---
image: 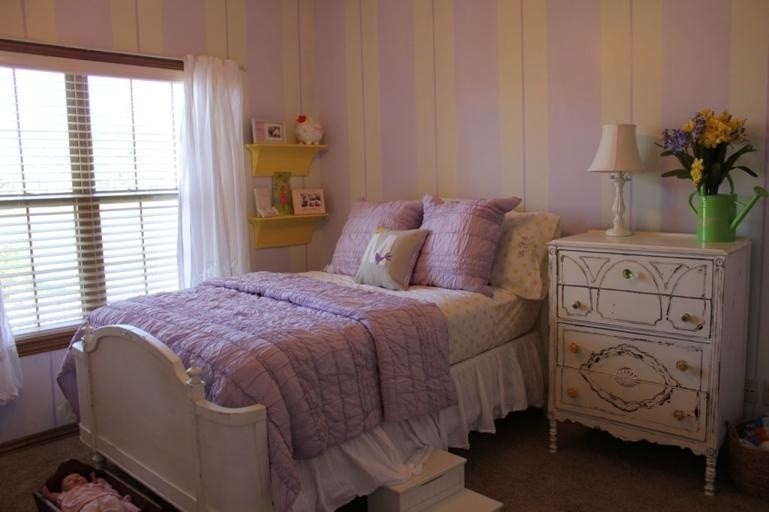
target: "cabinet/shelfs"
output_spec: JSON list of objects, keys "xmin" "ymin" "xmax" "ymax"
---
[{"xmin": 243, "ymin": 143, "xmax": 329, "ymax": 250}]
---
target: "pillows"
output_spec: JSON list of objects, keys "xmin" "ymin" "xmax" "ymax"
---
[{"xmin": 327, "ymin": 193, "xmax": 561, "ymax": 301}]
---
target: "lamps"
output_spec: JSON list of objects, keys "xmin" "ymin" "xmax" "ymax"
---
[{"xmin": 587, "ymin": 123, "xmax": 645, "ymax": 237}]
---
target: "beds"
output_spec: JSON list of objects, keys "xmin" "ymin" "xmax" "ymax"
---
[
  {"xmin": 72, "ymin": 270, "xmax": 549, "ymax": 512},
  {"xmin": 33, "ymin": 457, "xmax": 165, "ymax": 512}
]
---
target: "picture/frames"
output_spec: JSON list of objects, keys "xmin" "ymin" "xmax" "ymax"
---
[
  {"xmin": 291, "ymin": 189, "xmax": 326, "ymax": 215},
  {"xmin": 251, "ymin": 117, "xmax": 287, "ymax": 144}
]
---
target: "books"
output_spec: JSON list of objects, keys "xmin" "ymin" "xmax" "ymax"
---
[{"xmin": 253, "ymin": 187, "xmax": 272, "ymax": 219}]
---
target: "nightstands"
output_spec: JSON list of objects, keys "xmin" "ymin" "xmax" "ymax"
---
[{"xmin": 546, "ymin": 230, "xmax": 753, "ymax": 497}]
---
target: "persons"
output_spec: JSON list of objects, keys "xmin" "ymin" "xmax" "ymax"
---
[
  {"xmin": 39, "ymin": 470, "xmax": 142, "ymax": 512},
  {"xmin": 301, "ymin": 194, "xmax": 320, "ymax": 207},
  {"xmin": 272, "ymin": 126, "xmax": 280, "ymax": 137}
]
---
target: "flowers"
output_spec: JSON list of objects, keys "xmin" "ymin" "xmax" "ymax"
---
[{"xmin": 654, "ymin": 108, "xmax": 758, "ymax": 194}]
